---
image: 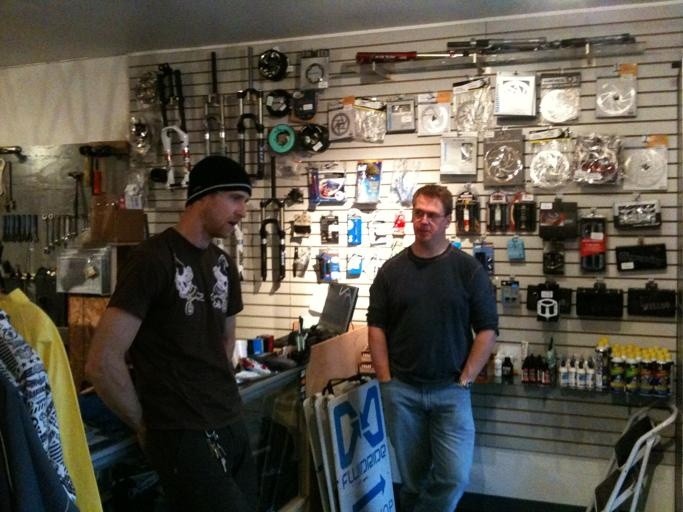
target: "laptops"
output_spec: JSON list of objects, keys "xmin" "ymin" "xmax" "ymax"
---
[{"xmin": 275, "ymin": 282, "xmax": 359, "ymax": 347}]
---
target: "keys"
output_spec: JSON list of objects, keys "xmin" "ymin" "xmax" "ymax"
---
[{"xmin": 205, "ymin": 428, "xmax": 228, "ymax": 473}]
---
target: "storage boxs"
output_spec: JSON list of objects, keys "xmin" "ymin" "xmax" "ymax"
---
[{"xmin": 89, "ymin": 208, "xmax": 143, "ymax": 242}]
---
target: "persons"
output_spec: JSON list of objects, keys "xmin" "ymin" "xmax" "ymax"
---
[
  {"xmin": 84, "ymin": 156, "xmax": 259, "ymax": 512},
  {"xmin": 365, "ymin": 185, "xmax": 499, "ymax": 512}
]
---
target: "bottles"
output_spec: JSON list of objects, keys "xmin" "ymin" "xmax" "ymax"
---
[
  {"xmin": 493, "ymin": 355, "xmax": 514, "ymax": 378},
  {"xmin": 519, "ymin": 347, "xmax": 594, "ymax": 392},
  {"xmin": 593, "ymin": 336, "xmax": 676, "ymax": 397},
  {"xmin": 295, "ymin": 333, "xmax": 304, "ymax": 351}
]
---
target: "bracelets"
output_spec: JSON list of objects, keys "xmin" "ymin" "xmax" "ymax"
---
[{"xmin": 461, "ymin": 380, "xmax": 473, "ymax": 390}]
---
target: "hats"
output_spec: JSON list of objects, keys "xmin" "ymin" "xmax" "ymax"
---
[{"xmin": 185, "ymin": 155, "xmax": 251, "ymax": 209}]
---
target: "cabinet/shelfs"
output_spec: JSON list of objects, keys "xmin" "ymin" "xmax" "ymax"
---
[{"xmin": 78, "ymin": 363, "xmax": 312, "ymax": 512}]
